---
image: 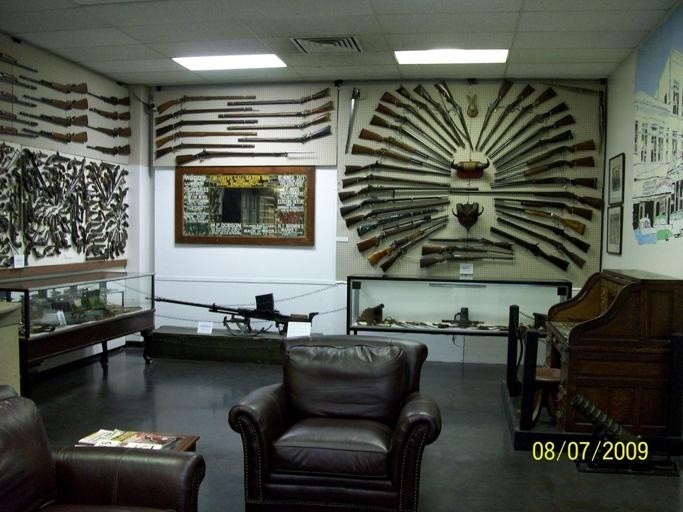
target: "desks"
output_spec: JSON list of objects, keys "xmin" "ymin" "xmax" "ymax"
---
[
  {"xmin": 152, "ymin": 429, "xmax": 200, "ymax": 456},
  {"xmin": 546, "ymin": 268, "xmax": 683, "ymax": 432}
]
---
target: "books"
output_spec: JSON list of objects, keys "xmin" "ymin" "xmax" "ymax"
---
[{"xmin": 75, "ymin": 429, "xmax": 185, "ymax": 450}]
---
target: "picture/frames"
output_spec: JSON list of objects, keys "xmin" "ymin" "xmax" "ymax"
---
[
  {"xmin": 171, "ymin": 166, "xmax": 317, "ymax": 251},
  {"xmin": 606, "ymin": 153, "xmax": 625, "ymax": 255}
]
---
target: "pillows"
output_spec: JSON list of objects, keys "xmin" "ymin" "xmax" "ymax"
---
[{"xmin": 273, "ymin": 416, "xmax": 396, "ymax": 474}]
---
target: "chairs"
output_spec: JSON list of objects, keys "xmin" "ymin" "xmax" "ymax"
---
[
  {"xmin": 227, "ymin": 332, "xmax": 444, "ymax": 512},
  {"xmin": 0, "ymin": 385, "xmax": 205, "ymax": 511}
]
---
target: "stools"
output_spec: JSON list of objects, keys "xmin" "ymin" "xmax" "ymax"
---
[{"xmin": 533, "ymin": 367, "xmax": 562, "ymax": 425}]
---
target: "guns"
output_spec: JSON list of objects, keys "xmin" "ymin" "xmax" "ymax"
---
[
  {"xmin": 145, "ymin": 292, "xmax": 319, "ymax": 333},
  {"xmin": 86, "ymin": 89, "xmax": 131, "ymax": 139},
  {"xmin": 155, "ymin": 85, "xmax": 334, "ymax": 165},
  {"xmin": 19, "ymin": 74, "xmax": 88, "ymax": 145},
  {"xmin": 0, "ymin": 51, "xmax": 36, "ymax": 139},
  {"xmin": 0, "ymin": 140, "xmax": 130, "ymax": 269}
]
